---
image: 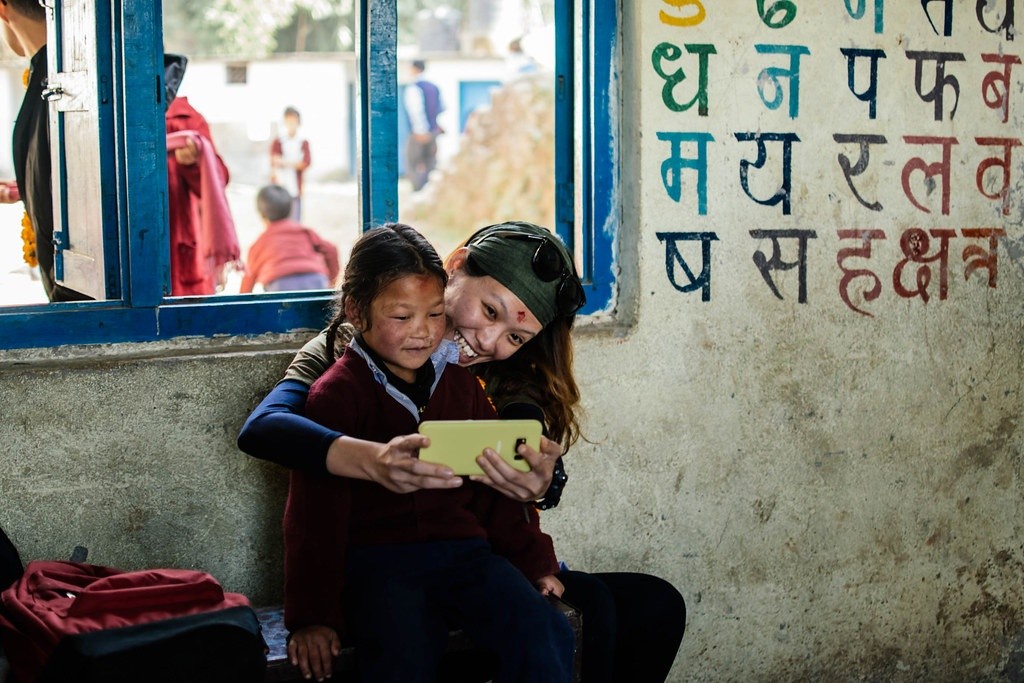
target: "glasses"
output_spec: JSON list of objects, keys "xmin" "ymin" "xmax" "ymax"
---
[{"xmin": 471, "ymin": 225, "xmax": 586, "ymax": 323}]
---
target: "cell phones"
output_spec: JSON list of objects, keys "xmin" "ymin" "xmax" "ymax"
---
[{"xmin": 417, "ymin": 419, "xmax": 542, "ymax": 476}]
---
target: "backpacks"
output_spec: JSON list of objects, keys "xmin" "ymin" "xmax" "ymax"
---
[{"xmin": 0, "ymin": 532, "xmax": 270, "ymax": 681}]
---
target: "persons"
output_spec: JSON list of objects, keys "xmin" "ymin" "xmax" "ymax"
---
[
  {"xmin": 0, "ymin": 1, "xmax": 90, "ymax": 307},
  {"xmin": 164, "ymin": 95, "xmax": 239, "ymax": 298},
  {"xmin": 403, "ymin": 59, "xmax": 445, "ymax": 192},
  {"xmin": 236, "ymin": 221, "xmax": 687, "ymax": 683},
  {"xmin": 285, "ymin": 223, "xmax": 578, "ymax": 683},
  {"xmin": 270, "ymin": 106, "xmax": 311, "ymax": 223},
  {"xmin": 238, "ymin": 184, "xmax": 339, "ymax": 296}
]
